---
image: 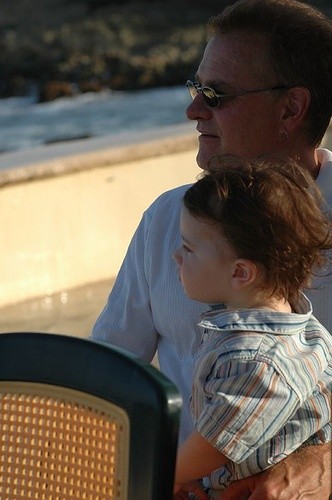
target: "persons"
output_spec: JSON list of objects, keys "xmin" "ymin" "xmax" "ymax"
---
[
  {"xmin": 87, "ymin": 1, "xmax": 332, "ymax": 500},
  {"xmin": 174, "ymin": 153, "xmax": 332, "ymax": 500}
]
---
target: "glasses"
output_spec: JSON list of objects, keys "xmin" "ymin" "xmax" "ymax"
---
[{"xmin": 184, "ymin": 80, "xmax": 320, "ymax": 110}]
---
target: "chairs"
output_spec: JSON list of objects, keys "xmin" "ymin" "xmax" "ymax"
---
[{"xmin": 0, "ymin": 332, "xmax": 182, "ymax": 500}]
